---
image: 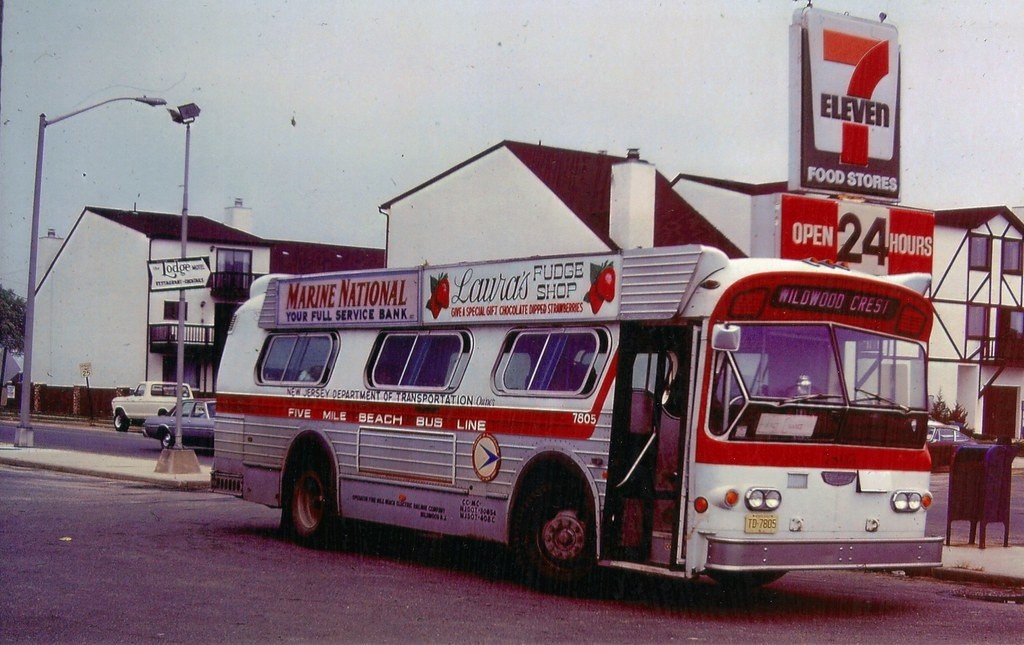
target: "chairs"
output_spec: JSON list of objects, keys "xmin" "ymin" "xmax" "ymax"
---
[{"xmin": 630, "ymin": 388, "xmax": 653, "ymax": 433}]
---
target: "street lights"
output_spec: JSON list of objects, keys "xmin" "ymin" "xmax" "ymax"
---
[
  {"xmin": 13, "ymin": 95, "xmax": 169, "ymax": 447},
  {"xmin": 165, "ymin": 101, "xmax": 205, "ymax": 448}
]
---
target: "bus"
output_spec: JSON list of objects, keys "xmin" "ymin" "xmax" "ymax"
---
[{"xmin": 207, "ymin": 246, "xmax": 944, "ymax": 593}]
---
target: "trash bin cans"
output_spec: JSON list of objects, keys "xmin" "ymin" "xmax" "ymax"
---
[
  {"xmin": 947, "ymin": 443, "xmax": 1020, "ymax": 548},
  {"xmin": 6, "ymin": 381, "xmax": 22, "ymax": 413}
]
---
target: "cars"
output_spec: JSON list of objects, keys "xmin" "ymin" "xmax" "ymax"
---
[
  {"xmin": 144, "ymin": 397, "xmax": 214, "ymax": 449},
  {"xmin": 927, "ymin": 423, "xmax": 980, "ymax": 471},
  {"xmin": 109, "ymin": 382, "xmax": 195, "ymax": 433}
]
